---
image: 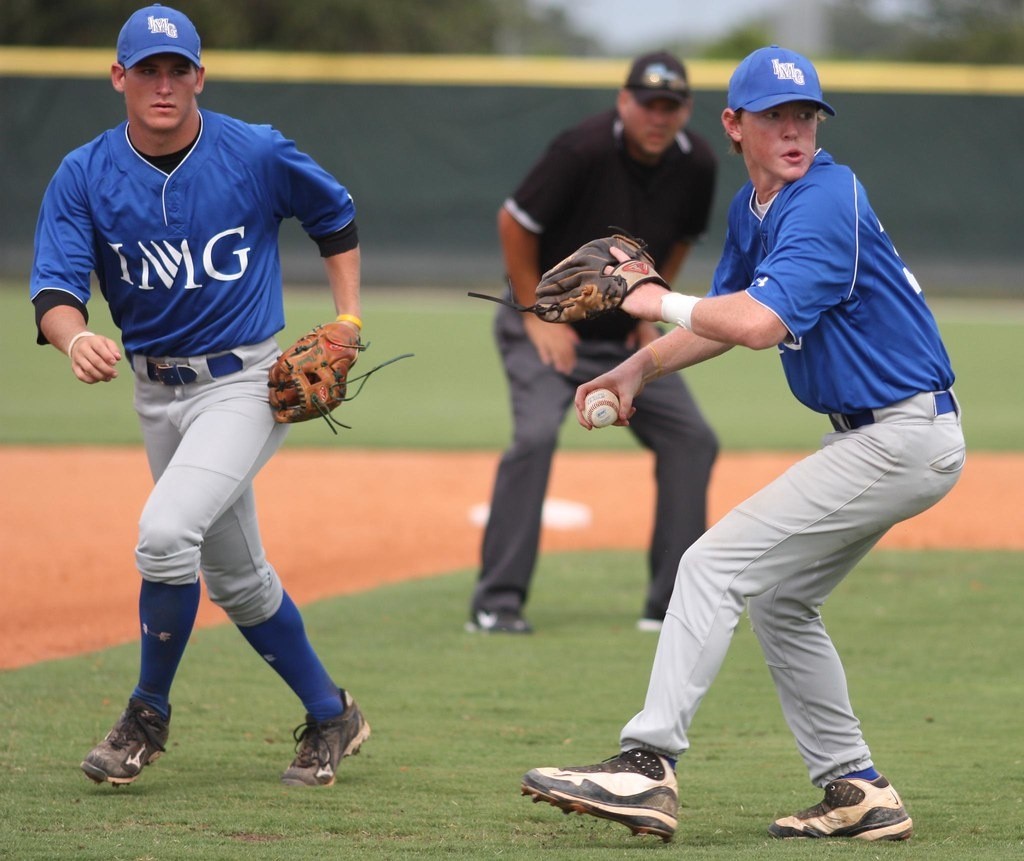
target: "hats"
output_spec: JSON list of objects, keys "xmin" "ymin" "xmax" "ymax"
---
[
  {"xmin": 117, "ymin": 3, "xmax": 201, "ymax": 70},
  {"xmin": 728, "ymin": 44, "xmax": 836, "ymax": 117},
  {"xmin": 624, "ymin": 51, "xmax": 690, "ymax": 109}
]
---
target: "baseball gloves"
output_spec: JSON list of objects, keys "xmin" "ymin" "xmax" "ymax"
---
[
  {"xmin": 266, "ymin": 323, "xmax": 360, "ymax": 424},
  {"xmin": 536, "ymin": 233, "xmax": 672, "ymax": 322}
]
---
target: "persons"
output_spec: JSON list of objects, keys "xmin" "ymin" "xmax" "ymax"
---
[
  {"xmin": 466, "ymin": 50, "xmax": 720, "ymax": 637},
  {"xmin": 29, "ymin": 2, "xmax": 371, "ymax": 786},
  {"xmin": 522, "ymin": 45, "xmax": 964, "ymax": 839}
]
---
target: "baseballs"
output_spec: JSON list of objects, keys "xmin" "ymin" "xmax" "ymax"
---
[{"xmin": 581, "ymin": 388, "xmax": 621, "ymax": 430}]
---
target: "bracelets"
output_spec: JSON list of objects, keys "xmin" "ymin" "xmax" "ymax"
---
[
  {"xmin": 67, "ymin": 331, "xmax": 96, "ymax": 360},
  {"xmin": 646, "ymin": 344, "xmax": 661, "ymax": 380},
  {"xmin": 335, "ymin": 314, "xmax": 362, "ymax": 331}
]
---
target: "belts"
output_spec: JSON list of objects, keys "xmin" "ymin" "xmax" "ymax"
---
[
  {"xmin": 131, "ymin": 352, "xmax": 242, "ymax": 386},
  {"xmin": 828, "ymin": 390, "xmax": 955, "ymax": 431}
]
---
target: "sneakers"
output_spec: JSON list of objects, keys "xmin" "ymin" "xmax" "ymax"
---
[
  {"xmin": 281, "ymin": 687, "xmax": 371, "ymax": 789},
  {"xmin": 520, "ymin": 749, "xmax": 679, "ymax": 843},
  {"xmin": 767, "ymin": 770, "xmax": 913, "ymax": 842},
  {"xmin": 80, "ymin": 696, "xmax": 172, "ymax": 788},
  {"xmin": 464, "ymin": 607, "xmax": 532, "ymax": 636},
  {"xmin": 638, "ymin": 605, "xmax": 664, "ymax": 632}
]
